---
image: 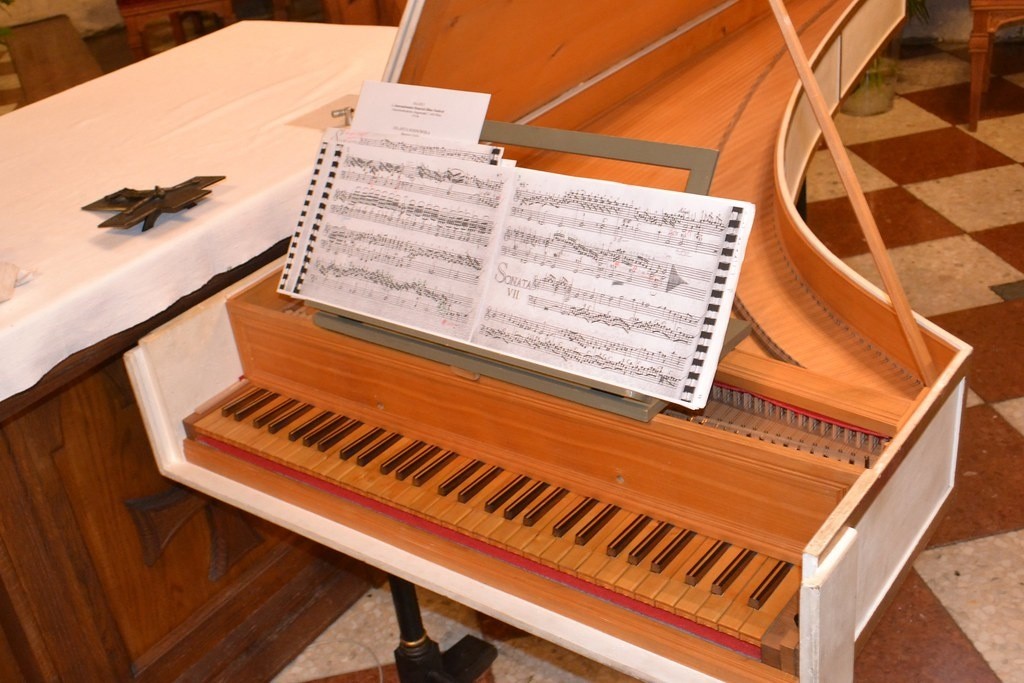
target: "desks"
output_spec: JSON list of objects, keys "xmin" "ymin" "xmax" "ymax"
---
[{"xmin": 0, "ymin": 16, "xmax": 403, "ymax": 682}]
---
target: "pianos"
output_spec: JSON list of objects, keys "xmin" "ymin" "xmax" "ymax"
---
[{"xmin": 122, "ymin": 0, "xmax": 973, "ymax": 683}]
